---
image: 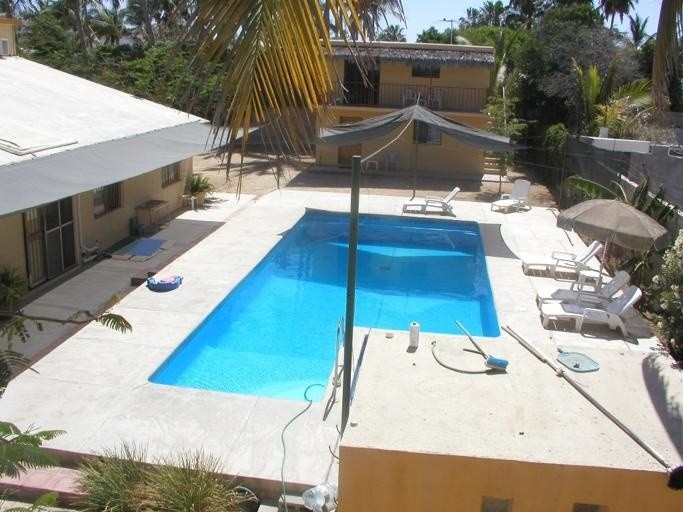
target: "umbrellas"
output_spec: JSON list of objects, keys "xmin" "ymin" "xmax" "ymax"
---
[{"xmin": 557, "ymin": 198, "xmax": 669, "ymax": 287}]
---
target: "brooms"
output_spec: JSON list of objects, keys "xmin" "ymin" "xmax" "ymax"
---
[{"xmin": 454, "ymin": 320, "xmax": 509, "ymax": 370}]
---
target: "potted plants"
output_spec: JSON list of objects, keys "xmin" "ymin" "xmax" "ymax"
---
[{"xmin": 189, "ymin": 175, "xmax": 212, "ymax": 207}]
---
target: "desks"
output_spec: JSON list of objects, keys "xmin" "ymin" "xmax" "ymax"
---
[
  {"xmin": 135, "ymin": 200, "xmax": 172, "ymax": 235},
  {"xmin": 577, "ymin": 270, "xmax": 603, "ymax": 292}
]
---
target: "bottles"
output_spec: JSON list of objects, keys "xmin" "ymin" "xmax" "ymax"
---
[{"xmin": 409, "ymin": 323, "xmax": 419, "ymax": 348}]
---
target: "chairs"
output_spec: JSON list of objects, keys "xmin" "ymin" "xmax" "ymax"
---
[
  {"xmin": 521, "ymin": 240, "xmax": 604, "ymax": 282},
  {"xmin": 491, "ymin": 179, "xmax": 532, "ymax": 213},
  {"xmin": 365, "ymin": 159, "xmax": 380, "ymax": 170},
  {"xmin": 539, "ymin": 285, "xmax": 643, "ymax": 336},
  {"xmin": 410, "ymin": 229, "xmax": 457, "ymax": 251},
  {"xmin": 536, "ymin": 270, "xmax": 631, "ymax": 310},
  {"xmin": 403, "ymin": 186, "xmax": 460, "ymax": 217},
  {"xmin": 386, "ymin": 150, "xmax": 401, "ymax": 172}
]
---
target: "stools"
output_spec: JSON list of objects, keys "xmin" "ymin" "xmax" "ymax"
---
[{"xmin": 178, "ymin": 194, "xmax": 199, "ymax": 210}]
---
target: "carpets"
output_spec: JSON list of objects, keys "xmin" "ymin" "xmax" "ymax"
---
[{"xmin": 104, "ymin": 232, "xmax": 167, "ymax": 257}]
